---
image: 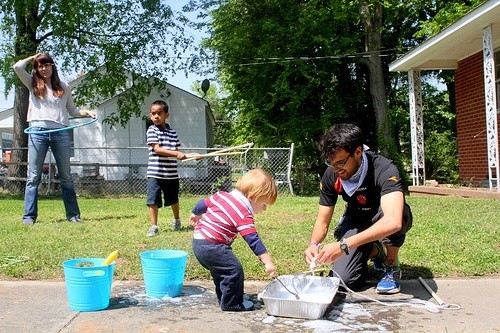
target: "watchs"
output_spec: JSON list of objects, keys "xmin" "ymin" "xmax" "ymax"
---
[{"xmin": 340, "ymin": 239, "xmax": 349, "ymax": 255}]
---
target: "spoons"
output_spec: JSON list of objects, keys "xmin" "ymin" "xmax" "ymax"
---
[{"xmin": 276, "ymin": 276, "xmax": 299, "ymax": 300}]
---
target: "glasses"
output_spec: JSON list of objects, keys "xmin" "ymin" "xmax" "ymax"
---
[
  {"xmin": 324, "ymin": 150, "xmax": 351, "ymax": 169},
  {"xmin": 36, "ymin": 63, "xmax": 55, "ymax": 68}
]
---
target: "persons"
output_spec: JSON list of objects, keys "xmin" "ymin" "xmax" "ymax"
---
[
  {"xmin": 189, "ymin": 168, "xmax": 278, "ymax": 311},
  {"xmin": 146, "ymin": 100, "xmax": 202, "ymax": 236},
  {"xmin": 304, "ymin": 123, "xmax": 413, "ymax": 294},
  {"xmin": 14, "ymin": 53, "xmax": 98, "ymax": 224}
]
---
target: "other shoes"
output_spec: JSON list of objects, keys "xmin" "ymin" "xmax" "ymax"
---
[
  {"xmin": 22, "ymin": 219, "xmax": 36, "ymax": 226},
  {"xmin": 370, "ymin": 241, "xmax": 386, "ymax": 277},
  {"xmin": 220, "ymin": 300, "xmax": 254, "ymax": 311},
  {"xmin": 70, "ymin": 215, "xmax": 82, "ymax": 223},
  {"xmin": 216, "ymin": 295, "xmax": 250, "ymax": 302},
  {"xmin": 376, "ymin": 263, "xmax": 403, "ymax": 293},
  {"xmin": 146, "ymin": 226, "xmax": 159, "ymax": 237},
  {"xmin": 173, "ymin": 222, "xmax": 181, "ymax": 230}
]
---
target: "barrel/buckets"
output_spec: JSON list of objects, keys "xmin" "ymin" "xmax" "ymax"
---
[
  {"xmin": 140, "ymin": 249, "xmax": 188, "ymax": 299},
  {"xmin": 62, "ymin": 257, "xmax": 115, "ymax": 312}
]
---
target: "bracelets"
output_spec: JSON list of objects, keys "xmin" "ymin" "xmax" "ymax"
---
[{"xmin": 310, "ymin": 243, "xmax": 321, "ymax": 248}]
